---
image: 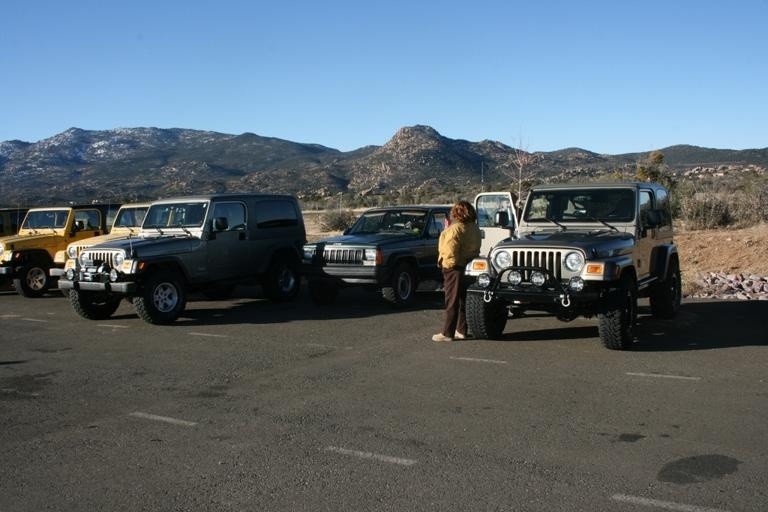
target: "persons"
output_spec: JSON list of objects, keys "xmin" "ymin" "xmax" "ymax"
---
[{"xmin": 432, "ymin": 200, "xmax": 482, "ymax": 343}]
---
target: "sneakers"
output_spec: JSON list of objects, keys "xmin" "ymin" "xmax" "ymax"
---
[
  {"xmin": 454, "ymin": 330, "xmax": 466, "ymax": 339},
  {"xmin": 432, "ymin": 333, "xmax": 453, "ymax": 342}
]
